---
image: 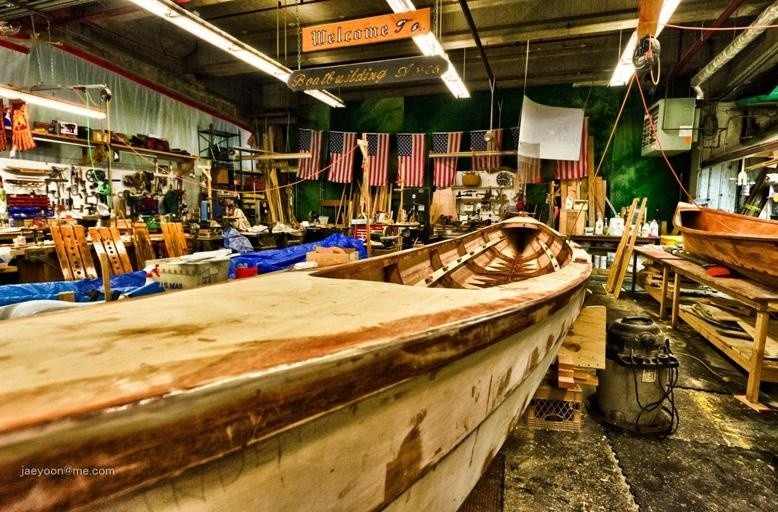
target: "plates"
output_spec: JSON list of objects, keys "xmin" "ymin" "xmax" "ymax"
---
[{"xmin": 497, "ymin": 173, "xmax": 510, "ymax": 185}]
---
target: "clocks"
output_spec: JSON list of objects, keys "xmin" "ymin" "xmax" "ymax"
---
[{"xmin": 496, "ymin": 172, "xmax": 511, "ymax": 186}]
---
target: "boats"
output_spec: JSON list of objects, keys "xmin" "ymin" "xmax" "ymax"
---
[
  {"xmin": 0, "ymin": 215, "xmax": 593, "ymax": 511},
  {"xmin": 672, "ymin": 202, "xmax": 778, "ymax": 289}
]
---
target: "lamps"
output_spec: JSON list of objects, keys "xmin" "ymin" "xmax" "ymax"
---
[
  {"xmin": 385, "ymin": 0, "xmax": 473, "ymax": 100},
  {"xmin": 128, "ymin": 0, "xmax": 347, "ymax": 109},
  {"xmin": 0, "ymin": 82, "xmax": 107, "ymax": 119},
  {"xmin": 608, "ymin": 0, "xmax": 682, "ymax": 88}
]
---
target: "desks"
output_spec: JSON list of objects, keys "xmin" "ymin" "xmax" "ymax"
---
[
  {"xmin": 662, "ymin": 247, "xmax": 778, "ymax": 403},
  {"xmin": 627, "ymin": 245, "xmax": 688, "ymax": 319},
  {"xmin": 571, "ymin": 235, "xmax": 660, "ymax": 292},
  {"xmin": 0, "ymin": 228, "xmax": 350, "ymax": 286}
]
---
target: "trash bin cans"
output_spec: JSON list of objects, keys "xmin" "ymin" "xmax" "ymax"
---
[{"xmin": 305, "ymin": 228, "xmax": 322, "ymax": 243}]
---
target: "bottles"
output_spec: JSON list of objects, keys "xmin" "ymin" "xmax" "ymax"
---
[
  {"xmin": 595, "ymin": 212, "xmax": 609, "ymax": 236},
  {"xmin": 642, "ymin": 218, "xmax": 658, "ymax": 237},
  {"xmin": 462, "ymin": 173, "xmax": 480, "ymax": 187}
]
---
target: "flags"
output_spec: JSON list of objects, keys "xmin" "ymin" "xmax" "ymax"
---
[
  {"xmin": 294, "ymin": 127, "xmax": 323, "ymax": 181},
  {"xmin": 396, "ymin": 132, "xmax": 426, "ymax": 186},
  {"xmin": 471, "ymin": 129, "xmax": 503, "ymax": 172},
  {"xmin": 555, "ymin": 117, "xmax": 590, "ymax": 182},
  {"xmin": 431, "ymin": 129, "xmax": 465, "ymax": 187},
  {"xmin": 509, "ymin": 126, "xmax": 543, "ymax": 185},
  {"xmin": 360, "ymin": 132, "xmax": 390, "ymax": 187},
  {"xmin": 326, "ymin": 130, "xmax": 356, "ymax": 184}
]
